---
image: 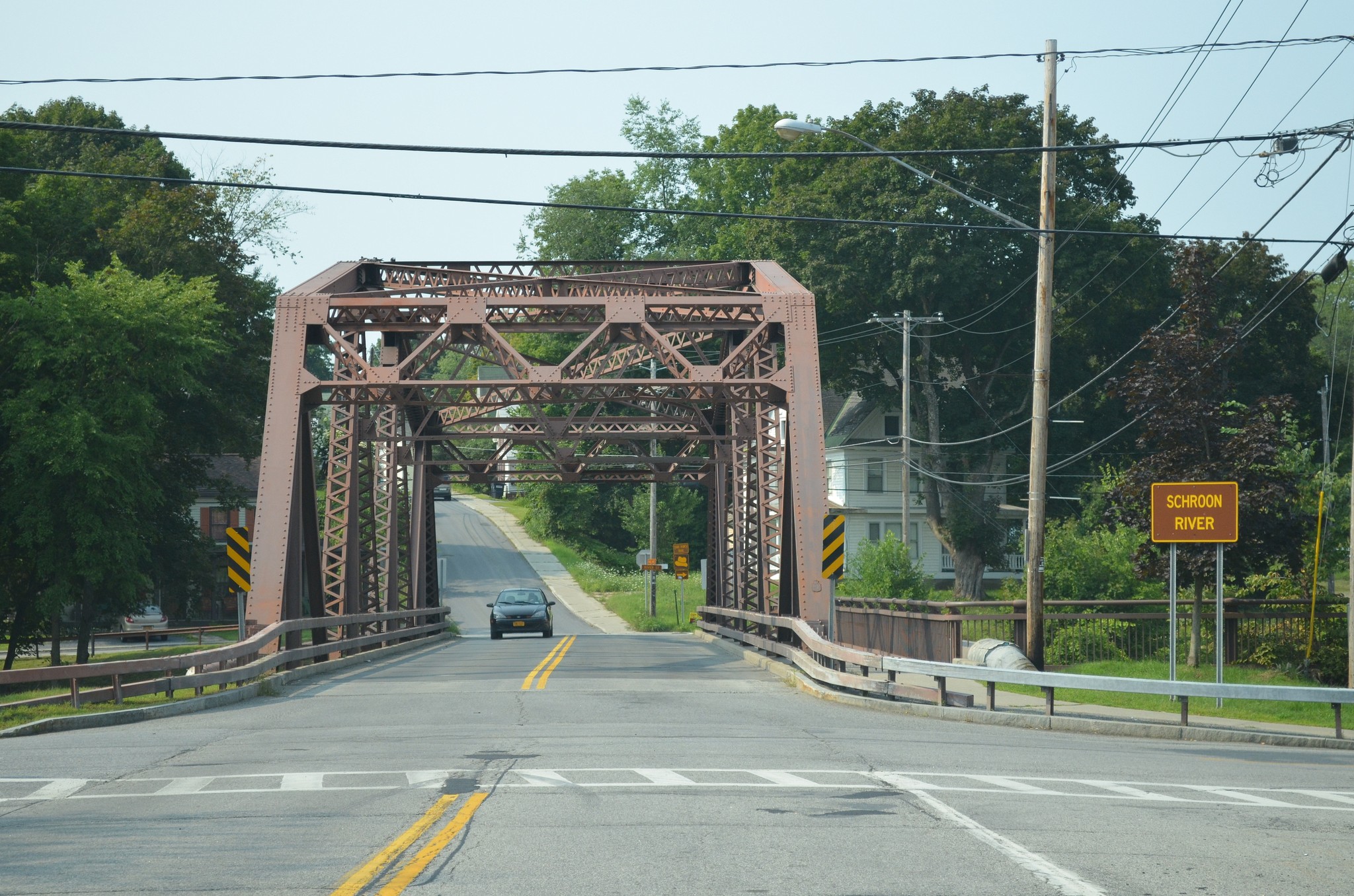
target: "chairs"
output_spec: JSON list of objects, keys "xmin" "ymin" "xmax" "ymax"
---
[
  {"xmin": 528, "ymin": 595, "xmax": 538, "ymax": 601},
  {"xmin": 506, "ymin": 596, "xmax": 515, "ymax": 603}
]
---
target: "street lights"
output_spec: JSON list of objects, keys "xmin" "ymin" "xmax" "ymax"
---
[{"xmin": 774, "ymin": 117, "xmax": 1058, "ymax": 670}]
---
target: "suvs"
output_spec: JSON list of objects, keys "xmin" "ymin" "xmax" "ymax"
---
[{"xmin": 434, "ymin": 475, "xmax": 452, "ymax": 501}]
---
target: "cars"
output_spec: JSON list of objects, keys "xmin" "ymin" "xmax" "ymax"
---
[
  {"xmin": 120, "ymin": 604, "xmax": 168, "ymax": 642},
  {"xmin": 486, "ymin": 587, "xmax": 555, "ymax": 639}
]
